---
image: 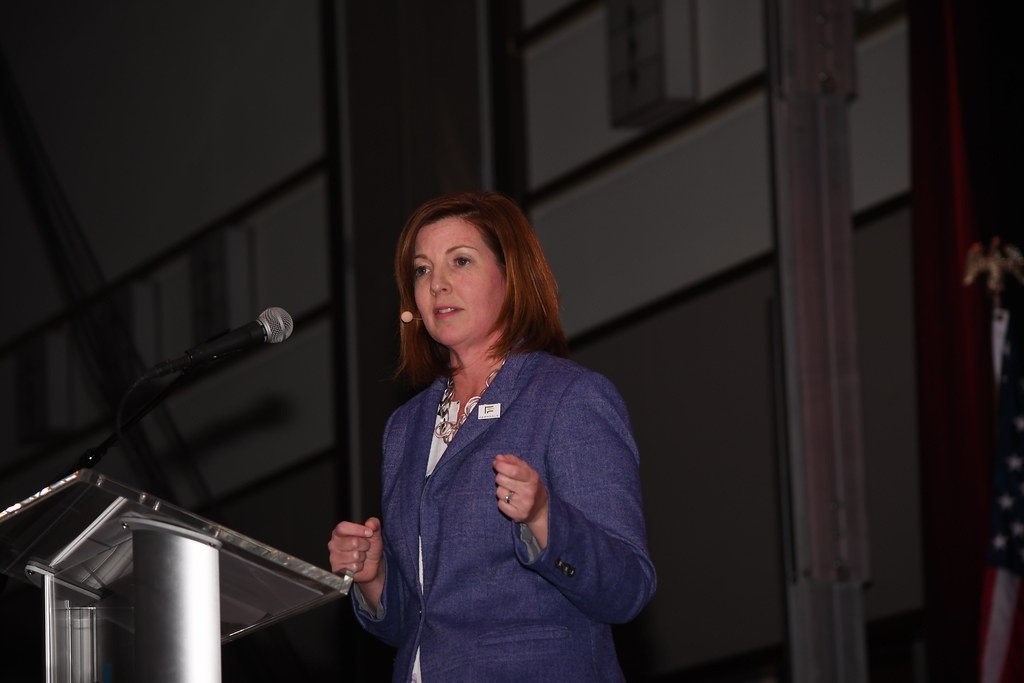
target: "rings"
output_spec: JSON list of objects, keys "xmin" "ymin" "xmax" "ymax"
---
[{"xmin": 504, "ymin": 491, "xmax": 516, "ymax": 504}]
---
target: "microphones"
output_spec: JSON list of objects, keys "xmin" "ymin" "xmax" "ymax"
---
[
  {"xmin": 401, "ymin": 311, "xmax": 423, "ymax": 322},
  {"xmin": 143, "ymin": 306, "xmax": 293, "ymax": 380}
]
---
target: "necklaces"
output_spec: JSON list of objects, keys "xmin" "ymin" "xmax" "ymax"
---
[{"xmin": 432, "ymin": 359, "xmax": 509, "ymax": 445}]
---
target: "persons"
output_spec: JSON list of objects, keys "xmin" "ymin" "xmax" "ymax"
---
[{"xmin": 328, "ymin": 190, "xmax": 662, "ymax": 683}]
